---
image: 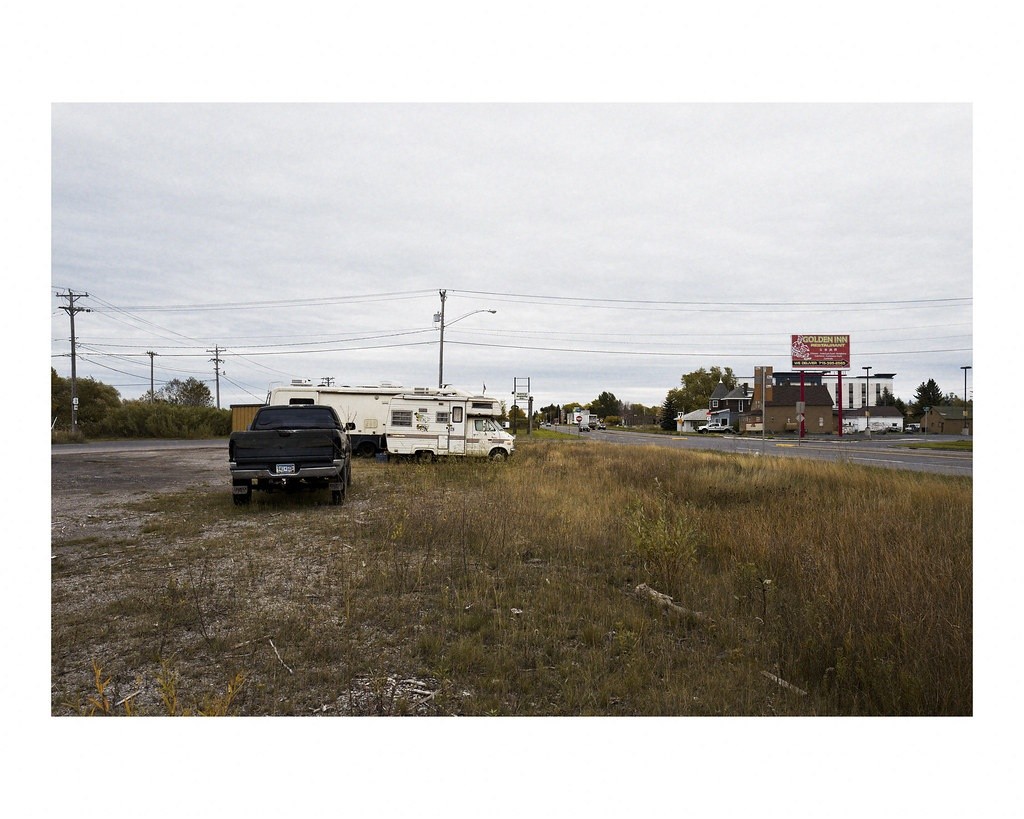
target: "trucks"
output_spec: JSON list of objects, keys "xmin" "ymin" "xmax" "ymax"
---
[
  {"xmin": 579, "ymin": 414, "xmax": 598, "ymax": 430},
  {"xmin": 268, "ymin": 385, "xmax": 474, "ymax": 459},
  {"xmin": 386, "ymin": 394, "xmax": 515, "ymax": 462}
]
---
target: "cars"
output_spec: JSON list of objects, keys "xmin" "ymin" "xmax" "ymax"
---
[
  {"xmin": 578, "ymin": 420, "xmax": 607, "ymax": 432},
  {"xmin": 905, "ymin": 423, "xmax": 920, "ymax": 431},
  {"xmin": 546, "ymin": 423, "xmax": 552, "ymax": 427}
]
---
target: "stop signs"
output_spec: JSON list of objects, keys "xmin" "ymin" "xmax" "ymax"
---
[{"xmin": 576, "ymin": 416, "xmax": 583, "ymax": 423}]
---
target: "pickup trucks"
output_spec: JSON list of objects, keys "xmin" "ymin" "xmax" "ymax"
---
[
  {"xmin": 229, "ymin": 405, "xmax": 356, "ymax": 506},
  {"xmin": 696, "ymin": 422, "xmax": 734, "ymax": 435}
]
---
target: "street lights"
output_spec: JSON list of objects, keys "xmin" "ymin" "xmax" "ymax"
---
[
  {"xmin": 438, "ymin": 310, "xmax": 498, "ymax": 388},
  {"xmin": 959, "ymin": 366, "xmax": 973, "ymax": 435},
  {"xmin": 862, "ymin": 366, "xmax": 873, "ymax": 440}
]
